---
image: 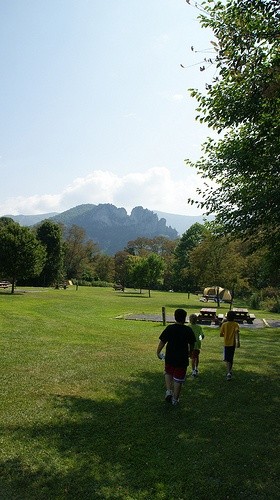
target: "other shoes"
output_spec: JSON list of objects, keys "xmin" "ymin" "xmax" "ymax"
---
[
  {"xmin": 165, "ymin": 390, "xmax": 179, "ymax": 406},
  {"xmin": 191, "ymin": 368, "xmax": 198, "ymax": 377},
  {"xmin": 226, "ymin": 371, "xmax": 232, "ymax": 381}
]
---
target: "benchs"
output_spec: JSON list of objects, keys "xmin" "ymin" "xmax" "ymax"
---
[{"xmin": 194, "ymin": 313, "xmax": 256, "ymax": 320}]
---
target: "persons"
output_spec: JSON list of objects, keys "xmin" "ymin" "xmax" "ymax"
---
[
  {"xmin": 157, "ymin": 309, "xmax": 197, "ymax": 406},
  {"xmin": 219, "ymin": 311, "xmax": 241, "ymax": 381},
  {"xmin": 186, "ymin": 314, "xmax": 205, "ymax": 377}
]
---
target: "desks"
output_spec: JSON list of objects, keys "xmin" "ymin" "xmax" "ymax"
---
[
  {"xmin": 200, "ymin": 308, "xmax": 217, "ymax": 325},
  {"xmin": 233, "ymin": 308, "xmax": 248, "ymax": 323}
]
